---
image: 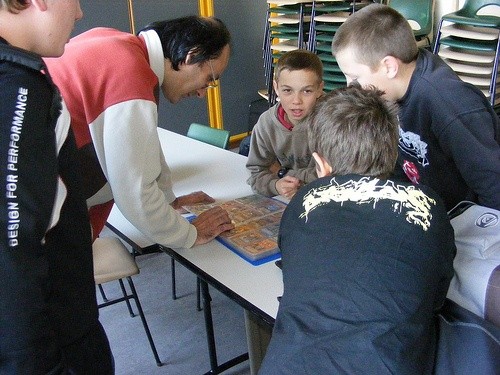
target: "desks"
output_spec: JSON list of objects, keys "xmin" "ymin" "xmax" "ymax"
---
[{"xmin": 105, "ymin": 127, "xmax": 291, "ymax": 375}]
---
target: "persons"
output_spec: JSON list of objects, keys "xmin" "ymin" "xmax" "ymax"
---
[
  {"xmin": 254, "ymin": 82, "xmax": 458, "ymax": 375},
  {"xmin": 332, "ymin": 3, "xmax": 500, "ymax": 276},
  {"xmin": 40, "ymin": 15, "xmax": 236, "ymax": 250},
  {"xmin": 246, "ymin": 48, "xmax": 334, "ymax": 198},
  {"xmin": 0, "ymin": 0, "xmax": 121, "ymax": 374}
]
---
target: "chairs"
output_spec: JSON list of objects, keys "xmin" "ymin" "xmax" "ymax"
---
[
  {"xmin": 94, "ymin": 235, "xmax": 162, "ymax": 367},
  {"xmin": 185, "ymin": 0, "xmax": 500, "ymax": 149}
]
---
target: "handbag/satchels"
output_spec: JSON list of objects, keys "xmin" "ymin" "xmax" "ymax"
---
[{"xmin": 443, "ymin": 200, "xmax": 500, "ymax": 321}]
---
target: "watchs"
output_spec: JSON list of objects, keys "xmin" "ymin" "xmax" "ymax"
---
[{"xmin": 278, "ymin": 166, "xmax": 292, "ymax": 179}]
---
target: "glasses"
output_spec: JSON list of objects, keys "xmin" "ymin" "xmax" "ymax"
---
[{"xmin": 204, "ymin": 55, "xmax": 220, "ymax": 87}]
